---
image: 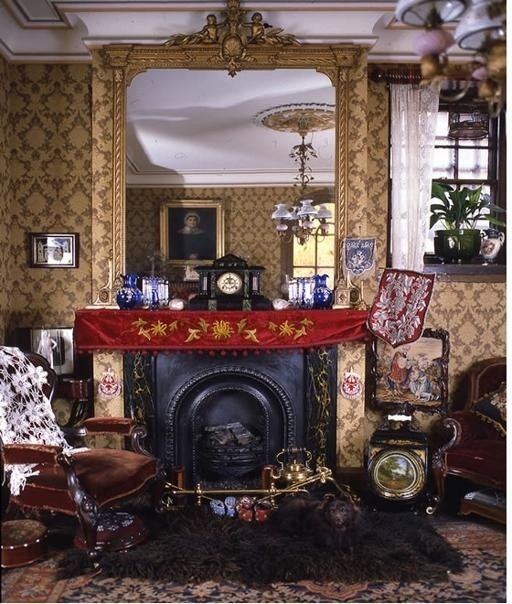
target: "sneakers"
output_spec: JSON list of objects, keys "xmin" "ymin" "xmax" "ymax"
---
[
  {"xmin": 236, "ymin": 495, "xmax": 254, "ymax": 522},
  {"xmin": 254, "ymin": 499, "xmax": 272, "ymax": 523},
  {"xmin": 209, "ymin": 499, "xmax": 226, "ymax": 516},
  {"xmin": 224, "ymin": 496, "xmax": 236, "ymax": 517}
]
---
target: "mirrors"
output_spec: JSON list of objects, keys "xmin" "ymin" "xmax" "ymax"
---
[{"xmin": 112, "ymin": 55, "xmax": 348, "ymax": 309}]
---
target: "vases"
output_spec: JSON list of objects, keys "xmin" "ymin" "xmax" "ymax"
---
[
  {"xmin": 115, "ymin": 274, "xmax": 135, "ymax": 308},
  {"xmin": 313, "ymin": 274, "xmax": 333, "ymax": 307},
  {"xmin": 480, "ymin": 228, "xmax": 505, "ymax": 263},
  {"xmin": 132, "ymin": 273, "xmax": 143, "ymax": 304}
]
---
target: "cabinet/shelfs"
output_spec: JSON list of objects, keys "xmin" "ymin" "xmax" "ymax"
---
[{"xmin": 87, "ymin": 308, "xmax": 366, "ymax": 493}]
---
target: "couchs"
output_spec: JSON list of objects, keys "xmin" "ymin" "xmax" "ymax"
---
[{"xmin": 426, "ymin": 355, "xmax": 507, "ymax": 528}]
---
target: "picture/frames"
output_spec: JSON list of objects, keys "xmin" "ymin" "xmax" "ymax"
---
[
  {"xmin": 366, "ymin": 327, "xmax": 451, "ymax": 414},
  {"xmin": 29, "ymin": 326, "xmax": 76, "ymax": 379},
  {"xmin": 28, "ymin": 232, "xmax": 79, "ymax": 269},
  {"xmin": 159, "ymin": 200, "xmax": 225, "ymax": 267}
]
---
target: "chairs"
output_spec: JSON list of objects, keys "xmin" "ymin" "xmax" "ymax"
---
[{"xmin": 0, "ymin": 344, "xmax": 162, "ymax": 601}]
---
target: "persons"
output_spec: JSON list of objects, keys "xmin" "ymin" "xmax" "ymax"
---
[
  {"xmin": 37, "ymin": 329, "xmax": 58, "ymax": 369},
  {"xmin": 172, "ymin": 211, "xmax": 210, "ymax": 259}
]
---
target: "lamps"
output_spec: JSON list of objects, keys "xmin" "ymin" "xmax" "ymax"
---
[
  {"xmin": 391, "ymin": 0, "xmax": 507, "ymax": 118},
  {"xmin": 262, "ymin": 109, "xmax": 336, "ymax": 240}
]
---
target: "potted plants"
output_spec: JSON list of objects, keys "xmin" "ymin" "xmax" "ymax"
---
[{"xmin": 429, "ymin": 181, "xmax": 506, "ymax": 263}]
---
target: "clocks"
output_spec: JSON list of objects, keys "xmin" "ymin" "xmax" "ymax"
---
[{"xmin": 187, "ymin": 252, "xmax": 274, "ymax": 310}]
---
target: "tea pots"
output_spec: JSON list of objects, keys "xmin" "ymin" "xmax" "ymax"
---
[{"xmin": 264, "ymin": 447, "xmax": 314, "ymax": 486}]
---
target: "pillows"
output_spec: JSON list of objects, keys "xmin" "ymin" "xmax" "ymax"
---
[{"xmin": 468, "ymin": 379, "xmax": 507, "ymax": 440}]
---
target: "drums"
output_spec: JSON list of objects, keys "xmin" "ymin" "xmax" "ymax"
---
[{"xmin": 0, "ymin": 519, "xmax": 50, "ymax": 569}]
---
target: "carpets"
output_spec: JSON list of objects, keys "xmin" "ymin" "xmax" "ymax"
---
[{"xmin": 53, "ymin": 495, "xmax": 471, "ymax": 587}]
